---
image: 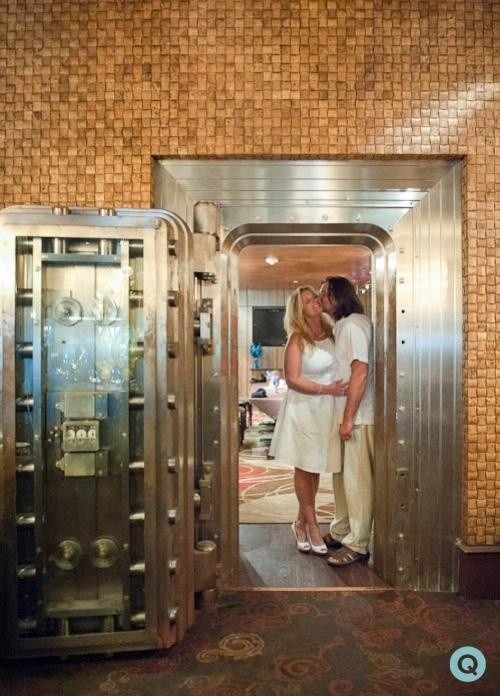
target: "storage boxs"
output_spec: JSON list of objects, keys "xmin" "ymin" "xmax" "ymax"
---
[{"xmin": 250, "ymin": 388, "xmax": 287, "ymax": 432}]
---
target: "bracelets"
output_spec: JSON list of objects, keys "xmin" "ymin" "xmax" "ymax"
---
[{"xmin": 317, "ymin": 383, "xmax": 322, "ymax": 396}]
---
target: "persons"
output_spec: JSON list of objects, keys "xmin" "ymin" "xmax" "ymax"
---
[
  {"xmin": 317, "ymin": 275, "xmax": 376, "ymax": 568},
  {"xmin": 265, "ymin": 283, "xmax": 351, "ymax": 557}
]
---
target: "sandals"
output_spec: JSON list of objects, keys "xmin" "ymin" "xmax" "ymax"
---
[
  {"xmin": 327, "ymin": 542, "xmax": 371, "ymax": 566},
  {"xmin": 321, "ymin": 533, "xmax": 343, "ymax": 548}
]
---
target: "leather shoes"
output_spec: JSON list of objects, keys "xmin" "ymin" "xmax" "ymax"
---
[
  {"xmin": 305, "ymin": 524, "xmax": 328, "ymax": 556},
  {"xmin": 292, "ymin": 521, "xmax": 311, "ymax": 552}
]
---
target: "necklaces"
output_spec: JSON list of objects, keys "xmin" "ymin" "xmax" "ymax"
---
[{"xmin": 313, "ymin": 329, "xmax": 328, "ymax": 340}]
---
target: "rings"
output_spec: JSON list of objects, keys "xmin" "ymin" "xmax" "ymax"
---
[{"xmin": 343, "ymin": 390, "xmax": 346, "ymax": 395}]
